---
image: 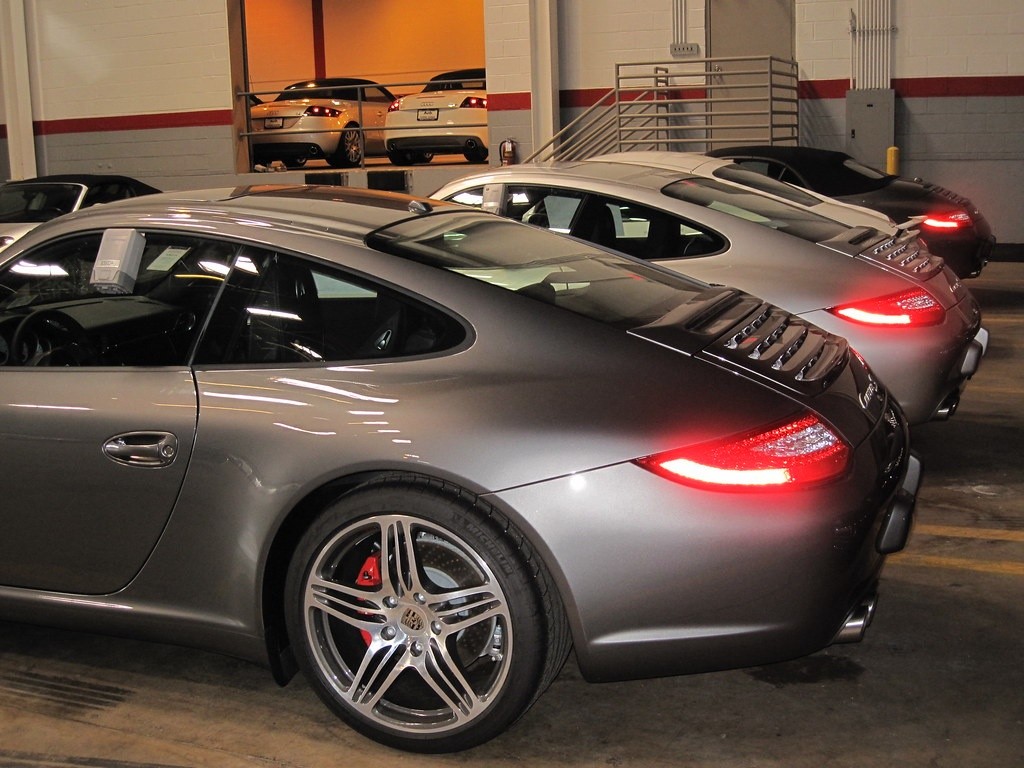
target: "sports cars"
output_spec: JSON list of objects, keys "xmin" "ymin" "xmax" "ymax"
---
[
  {"xmin": 704, "ymin": 140, "xmax": 993, "ymax": 281},
  {"xmin": 0, "ymin": 184, "xmax": 921, "ymax": 755},
  {"xmin": 0, "ymin": 172, "xmax": 164, "ymax": 263},
  {"xmin": 430, "ymin": 160, "xmax": 988, "ymax": 421},
  {"xmin": 381, "ymin": 64, "xmax": 488, "ymax": 163},
  {"xmin": 595, "ymin": 147, "xmax": 899, "ymax": 236},
  {"xmin": 250, "ymin": 75, "xmax": 396, "ymax": 170}
]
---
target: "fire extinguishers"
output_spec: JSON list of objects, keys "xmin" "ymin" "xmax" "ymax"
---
[{"xmin": 498, "ymin": 136, "xmax": 519, "ymax": 167}]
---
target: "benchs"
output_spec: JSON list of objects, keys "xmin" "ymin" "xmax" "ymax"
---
[{"xmin": 305, "ymin": 290, "xmax": 407, "ymax": 364}]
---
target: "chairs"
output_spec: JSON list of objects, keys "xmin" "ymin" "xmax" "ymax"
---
[{"xmin": 579, "ymin": 205, "xmax": 619, "ymax": 249}]
